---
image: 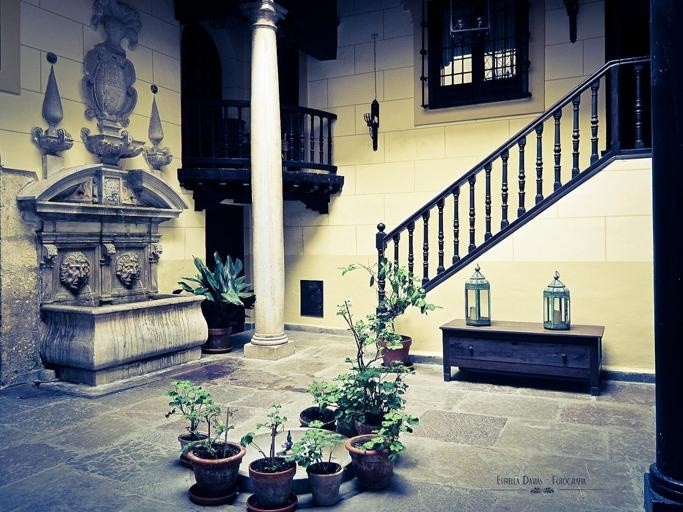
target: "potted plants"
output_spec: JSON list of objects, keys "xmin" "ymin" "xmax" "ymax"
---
[
  {"xmin": 285, "ymin": 428, "xmax": 344, "ymax": 506},
  {"xmin": 299, "ymin": 381, "xmax": 344, "ymax": 431},
  {"xmin": 345, "ymin": 408, "xmax": 419, "ymax": 482},
  {"xmin": 181, "ymin": 405, "xmax": 246, "ymax": 506},
  {"xmin": 338, "ymin": 258, "xmax": 443, "ymax": 367},
  {"xmin": 164, "ymin": 380, "xmax": 221, "ymax": 466},
  {"xmin": 172, "ymin": 252, "xmax": 256, "ymax": 354},
  {"xmin": 336, "ymin": 300, "xmax": 416, "ymax": 435},
  {"xmin": 240, "ymin": 403, "xmax": 298, "ymax": 512}
]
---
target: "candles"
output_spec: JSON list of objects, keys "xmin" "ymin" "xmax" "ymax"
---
[
  {"xmin": 470, "ymin": 307, "xmax": 476, "ymax": 320},
  {"xmin": 554, "ymin": 310, "xmax": 561, "ymax": 324}
]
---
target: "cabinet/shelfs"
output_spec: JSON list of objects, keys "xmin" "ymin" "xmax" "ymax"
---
[{"xmin": 439, "ymin": 319, "xmax": 605, "ymax": 395}]
